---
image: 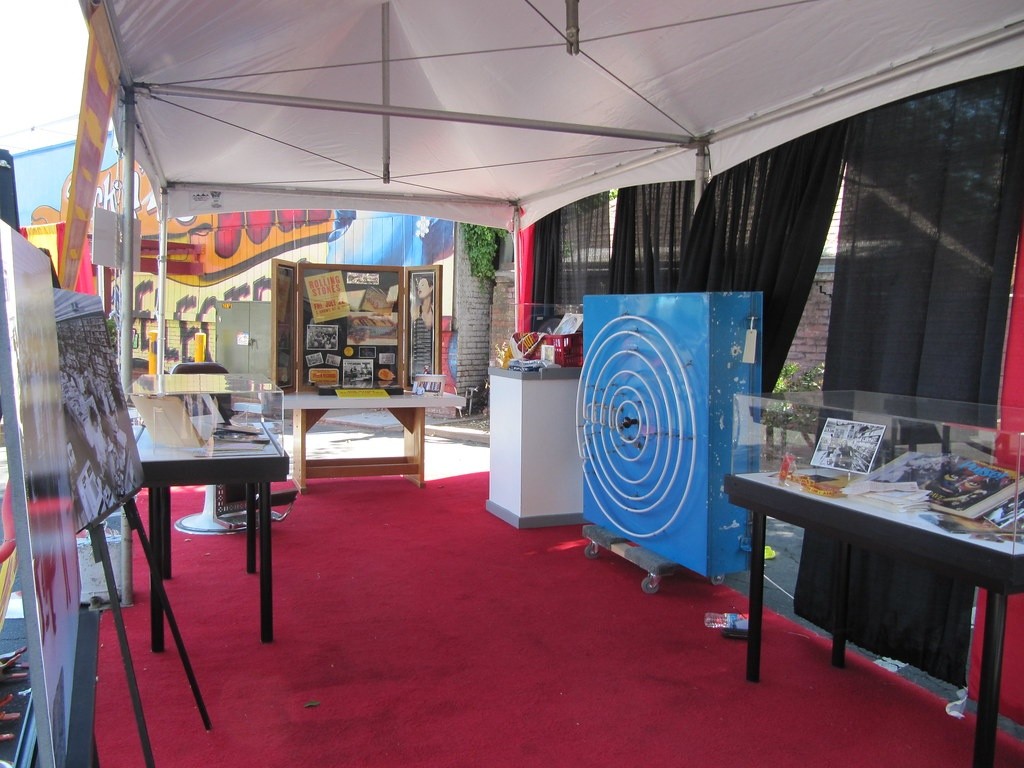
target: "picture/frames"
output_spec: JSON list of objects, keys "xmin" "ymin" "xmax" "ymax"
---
[{"xmin": 412, "ymin": 374, "xmax": 447, "ymax": 399}]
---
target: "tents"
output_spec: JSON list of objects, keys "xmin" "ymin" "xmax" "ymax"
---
[{"xmin": 80, "ymin": 0, "xmax": 1024, "ymax": 603}]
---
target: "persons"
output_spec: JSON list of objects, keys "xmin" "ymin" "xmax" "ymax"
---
[
  {"xmin": 417, "ymin": 383, "xmax": 425, "ymax": 395},
  {"xmin": 838, "ymin": 440, "xmax": 859, "ymax": 470},
  {"xmin": 412, "ymin": 277, "xmax": 434, "ymax": 375}
]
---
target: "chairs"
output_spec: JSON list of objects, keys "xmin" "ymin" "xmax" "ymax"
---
[{"xmin": 175, "ymin": 362, "xmax": 298, "ymax": 535}]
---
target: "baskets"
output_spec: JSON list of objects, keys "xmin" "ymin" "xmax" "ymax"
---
[{"xmin": 527, "ymin": 331, "xmax": 583, "ymax": 367}]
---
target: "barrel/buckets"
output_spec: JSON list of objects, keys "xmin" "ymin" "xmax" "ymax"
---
[{"xmin": 415, "ymin": 374, "xmax": 446, "ymax": 399}]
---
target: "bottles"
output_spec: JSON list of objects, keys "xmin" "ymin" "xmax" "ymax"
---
[{"xmin": 703, "ymin": 612, "xmax": 749, "ymax": 630}]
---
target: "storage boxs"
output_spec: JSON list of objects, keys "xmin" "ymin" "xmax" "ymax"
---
[
  {"xmin": 319, "ymin": 387, "xmax": 340, "ymax": 395},
  {"xmin": 526, "ymin": 331, "xmax": 583, "ymax": 367},
  {"xmin": 384, "ymin": 387, "xmax": 404, "ymax": 395}
]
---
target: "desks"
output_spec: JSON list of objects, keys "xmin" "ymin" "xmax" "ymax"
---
[{"xmin": 283, "ymin": 390, "xmax": 467, "ymax": 495}]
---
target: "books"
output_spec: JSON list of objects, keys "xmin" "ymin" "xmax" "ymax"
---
[{"xmin": 864, "ymin": 450, "xmax": 1024, "ymax": 529}]
